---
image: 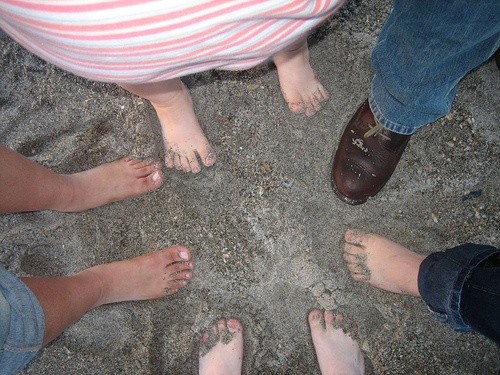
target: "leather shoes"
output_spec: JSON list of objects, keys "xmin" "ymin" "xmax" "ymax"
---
[{"xmin": 330, "ymin": 98, "xmax": 411, "ymax": 206}]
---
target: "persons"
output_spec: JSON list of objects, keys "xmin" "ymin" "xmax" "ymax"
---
[
  {"xmin": 341, "ymin": 228, "xmax": 500, "ymax": 345},
  {"xmin": 0, "ymin": 142, "xmax": 198, "ymax": 375},
  {"xmin": 0, "ymin": 0, "xmax": 347, "ymax": 174},
  {"xmin": 198, "ymin": 308, "xmax": 366, "ymax": 375},
  {"xmin": 331, "ymin": 0, "xmax": 500, "ymax": 206}
]
